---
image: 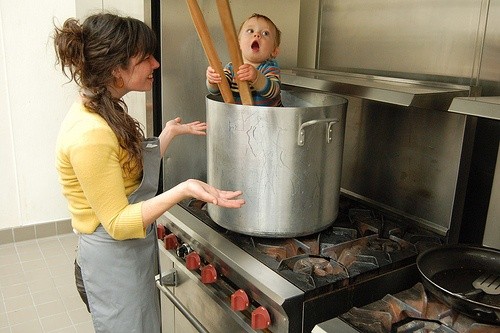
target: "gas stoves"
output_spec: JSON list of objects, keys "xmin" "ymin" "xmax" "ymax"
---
[
  {"xmin": 156, "ymin": 173, "xmax": 449, "ymax": 333},
  {"xmin": 310, "ymin": 281, "xmax": 500, "ymax": 333}
]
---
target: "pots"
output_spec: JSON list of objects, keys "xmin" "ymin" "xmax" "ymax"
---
[
  {"xmin": 205, "ymin": 84, "xmax": 348, "ymax": 238},
  {"xmin": 417, "ymin": 241, "xmax": 500, "ymax": 326}
]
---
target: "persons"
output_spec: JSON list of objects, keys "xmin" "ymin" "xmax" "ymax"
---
[
  {"xmin": 204, "ymin": 13, "xmax": 285, "ymax": 108},
  {"xmin": 47, "ymin": 12, "xmax": 246, "ymax": 333}
]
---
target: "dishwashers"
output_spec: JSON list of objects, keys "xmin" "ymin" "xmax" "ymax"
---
[{"xmin": 156, "ymin": 239, "xmax": 269, "ymax": 333}]
